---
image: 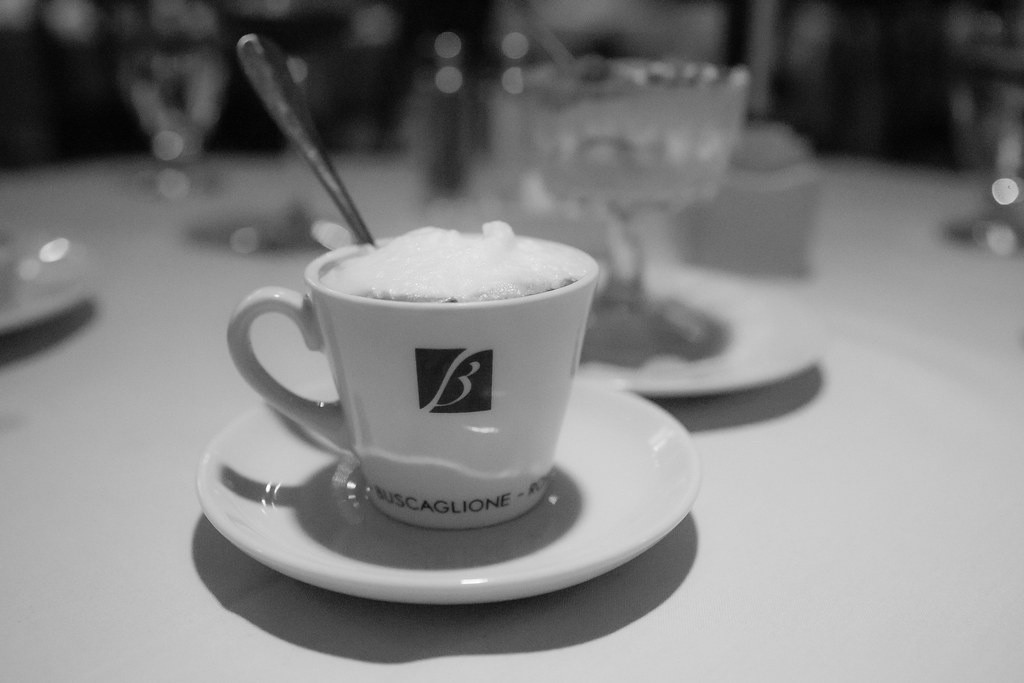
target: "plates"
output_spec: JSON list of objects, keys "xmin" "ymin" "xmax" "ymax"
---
[
  {"xmin": 0, "ymin": 239, "xmax": 97, "ymax": 334},
  {"xmin": 576, "ymin": 263, "xmax": 832, "ymax": 395},
  {"xmin": 197, "ymin": 382, "xmax": 702, "ymax": 605}
]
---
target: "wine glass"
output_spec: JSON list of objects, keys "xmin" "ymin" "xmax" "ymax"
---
[
  {"xmin": 506, "ymin": 57, "xmax": 751, "ymax": 366},
  {"xmin": 121, "ymin": 26, "xmax": 231, "ymax": 202}
]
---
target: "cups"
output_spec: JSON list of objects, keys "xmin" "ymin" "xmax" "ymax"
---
[{"xmin": 227, "ymin": 232, "xmax": 599, "ymax": 528}]
